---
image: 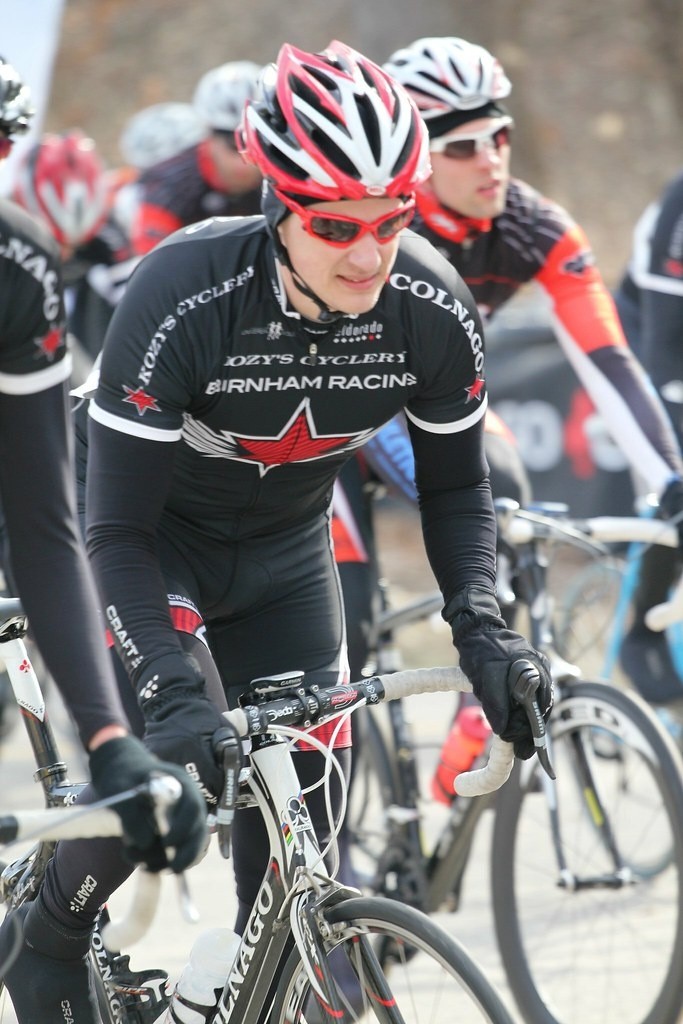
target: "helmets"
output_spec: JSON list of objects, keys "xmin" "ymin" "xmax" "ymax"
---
[
  {"xmin": 13, "ymin": 130, "xmax": 106, "ymax": 254},
  {"xmin": 196, "ymin": 61, "xmax": 262, "ymax": 129},
  {"xmin": 235, "ymin": 41, "xmax": 434, "ymax": 199},
  {"xmin": 119, "ymin": 102, "xmax": 210, "ymax": 167},
  {"xmin": 0, "ymin": 56, "xmax": 35, "ymax": 134},
  {"xmin": 382, "ymin": 35, "xmax": 512, "ymax": 118}
]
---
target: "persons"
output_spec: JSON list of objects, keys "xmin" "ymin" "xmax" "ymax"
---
[
  {"xmin": 330, "ymin": 37, "xmax": 680, "ymax": 683},
  {"xmin": 0, "ymin": 61, "xmax": 262, "ymax": 748},
  {"xmin": 565, "ymin": 173, "xmax": 683, "ymax": 706},
  {"xmin": 0, "ymin": 78, "xmax": 210, "ymax": 875},
  {"xmin": 0, "ymin": 39, "xmax": 555, "ymax": 1024}
]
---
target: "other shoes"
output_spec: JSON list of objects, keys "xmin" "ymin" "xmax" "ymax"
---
[{"xmin": 0, "ymin": 895, "xmax": 116, "ymax": 1024}]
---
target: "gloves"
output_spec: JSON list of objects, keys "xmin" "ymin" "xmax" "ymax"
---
[
  {"xmin": 487, "ymin": 512, "xmax": 550, "ymax": 606},
  {"xmin": 133, "ymin": 651, "xmax": 245, "ymax": 813},
  {"xmin": 441, "ymin": 584, "xmax": 555, "ymax": 760},
  {"xmin": 90, "ymin": 736, "xmax": 212, "ymax": 874}
]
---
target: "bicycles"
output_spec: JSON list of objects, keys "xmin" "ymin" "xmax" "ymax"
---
[{"xmin": 0, "ymin": 494, "xmax": 683, "ymax": 1024}]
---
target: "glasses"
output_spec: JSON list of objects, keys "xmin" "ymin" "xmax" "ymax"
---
[
  {"xmin": 430, "ymin": 115, "xmax": 514, "ymax": 160},
  {"xmin": 216, "ymin": 129, "xmax": 247, "ymax": 155},
  {"xmin": 266, "ymin": 182, "xmax": 417, "ymax": 245}
]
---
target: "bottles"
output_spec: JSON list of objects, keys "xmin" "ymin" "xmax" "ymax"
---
[
  {"xmin": 164, "ymin": 930, "xmax": 241, "ymax": 1023},
  {"xmin": 433, "ymin": 706, "xmax": 493, "ymax": 807}
]
